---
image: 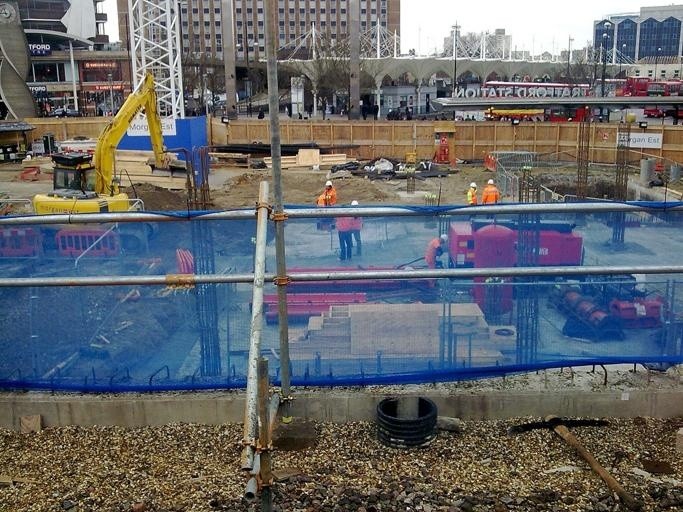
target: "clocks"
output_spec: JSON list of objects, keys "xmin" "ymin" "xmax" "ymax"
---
[{"xmin": 0, "ymin": 2, "xmax": 17, "ymax": 24}]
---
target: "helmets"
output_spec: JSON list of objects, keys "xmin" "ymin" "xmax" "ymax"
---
[
  {"xmin": 470, "ymin": 183, "xmax": 477, "ymax": 188},
  {"xmin": 352, "ymin": 200, "xmax": 358, "ymax": 206},
  {"xmin": 325, "ymin": 181, "xmax": 333, "ymax": 187},
  {"xmin": 441, "ymin": 234, "xmax": 448, "ymax": 242},
  {"xmin": 488, "ymin": 179, "xmax": 494, "ymax": 184}
]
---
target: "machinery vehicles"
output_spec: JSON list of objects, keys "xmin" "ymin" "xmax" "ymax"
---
[
  {"xmin": 33, "ymin": 69, "xmax": 192, "ymax": 253},
  {"xmin": 93, "ymin": 82, "xmax": 238, "ymax": 116}
]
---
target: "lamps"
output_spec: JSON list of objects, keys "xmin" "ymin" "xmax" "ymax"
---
[
  {"xmin": 512, "ymin": 119, "xmax": 519, "ymax": 125},
  {"xmin": 221, "ymin": 116, "xmax": 229, "ymax": 123},
  {"xmin": 639, "ymin": 122, "xmax": 648, "ymax": 128}
]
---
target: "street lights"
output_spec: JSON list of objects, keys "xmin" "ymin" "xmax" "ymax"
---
[
  {"xmin": 107, "ymin": 71, "xmax": 113, "ymax": 115},
  {"xmin": 201, "ymin": 72, "xmax": 209, "ymax": 118},
  {"xmin": 235, "ymin": 21, "xmax": 258, "ymax": 117},
  {"xmin": 619, "ymin": 43, "xmax": 626, "ymax": 73},
  {"xmin": 451, "ymin": 21, "xmax": 460, "ymax": 93},
  {"xmin": 601, "ymin": 21, "xmax": 611, "ymax": 98},
  {"xmin": 654, "ymin": 47, "xmax": 660, "ymax": 79}
]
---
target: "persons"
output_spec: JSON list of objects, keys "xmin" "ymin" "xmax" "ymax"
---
[
  {"xmin": 297, "ymin": 112, "xmax": 302, "ymax": 120},
  {"xmin": 484, "ymin": 114, "xmax": 549, "ymax": 123},
  {"xmin": 316, "ymin": 181, "xmax": 362, "ymax": 261},
  {"xmin": 425, "ymin": 234, "xmax": 449, "ymax": 288},
  {"xmin": 361, "ymin": 103, "xmax": 413, "ymax": 121},
  {"xmin": 61, "ymin": 107, "xmax": 120, "ymax": 116},
  {"xmin": 467, "ymin": 179, "xmax": 500, "ymax": 221},
  {"xmin": 421, "ymin": 113, "xmax": 477, "ymax": 122},
  {"xmin": 257, "ymin": 107, "xmax": 265, "ymax": 119},
  {"xmin": 307, "ymin": 104, "xmax": 313, "ymax": 118},
  {"xmin": 286, "ymin": 103, "xmax": 293, "ymax": 117},
  {"xmin": 184, "ymin": 105, "xmax": 216, "ymax": 118},
  {"xmin": 330, "ymin": 104, "xmax": 347, "ymax": 115}
]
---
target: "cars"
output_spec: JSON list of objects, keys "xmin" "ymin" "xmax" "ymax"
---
[{"xmin": 48, "ymin": 108, "xmax": 78, "ymax": 118}]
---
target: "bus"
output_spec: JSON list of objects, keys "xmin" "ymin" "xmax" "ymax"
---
[
  {"xmin": 480, "ymin": 80, "xmax": 591, "ymax": 124},
  {"xmin": 591, "ymin": 76, "xmax": 682, "ymax": 121}
]
